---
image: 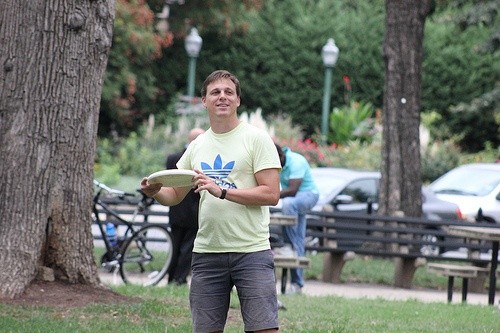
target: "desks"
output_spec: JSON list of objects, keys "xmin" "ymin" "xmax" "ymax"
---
[{"xmin": 449, "ymin": 225, "xmax": 500, "ymax": 305}]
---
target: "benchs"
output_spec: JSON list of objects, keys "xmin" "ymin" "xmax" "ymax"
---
[
  {"xmin": 427, "ymin": 262, "xmax": 477, "ymax": 303},
  {"xmin": 274, "ymin": 256, "xmax": 309, "ymax": 295},
  {"xmin": 93, "ymin": 198, "xmax": 500, "ymax": 294}
]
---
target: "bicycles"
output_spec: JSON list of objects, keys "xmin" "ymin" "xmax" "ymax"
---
[{"xmin": 90, "ymin": 177, "xmax": 175, "ymax": 289}]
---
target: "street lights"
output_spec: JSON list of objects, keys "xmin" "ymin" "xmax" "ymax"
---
[
  {"xmin": 321, "ymin": 37, "xmax": 339, "ymax": 147},
  {"xmin": 183, "ymin": 26, "xmax": 202, "ymax": 131}
]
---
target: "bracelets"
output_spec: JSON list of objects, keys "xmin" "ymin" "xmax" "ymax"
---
[{"xmin": 219, "ymin": 185, "xmax": 227, "ymax": 199}]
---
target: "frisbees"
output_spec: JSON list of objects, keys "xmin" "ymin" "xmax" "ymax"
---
[{"xmin": 147, "ymin": 169, "xmax": 199, "ymax": 188}]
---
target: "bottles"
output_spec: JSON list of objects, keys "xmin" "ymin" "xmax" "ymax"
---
[{"xmin": 106, "ymin": 222, "xmax": 118, "ymax": 251}]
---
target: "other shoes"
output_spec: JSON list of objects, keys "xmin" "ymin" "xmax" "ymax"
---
[
  {"xmin": 284, "ymin": 282, "xmax": 303, "ymax": 295},
  {"xmin": 274, "ymin": 245, "xmax": 298, "ymax": 256}
]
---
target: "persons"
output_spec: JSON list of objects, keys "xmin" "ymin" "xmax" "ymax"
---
[
  {"xmin": 120, "ymin": 81, "xmax": 155, "ymax": 141},
  {"xmin": 165, "ymin": 129, "xmax": 207, "ymax": 286},
  {"xmin": 274, "ymin": 143, "xmax": 318, "ymax": 292},
  {"xmin": 141, "ymin": 70, "xmax": 282, "ymax": 333}
]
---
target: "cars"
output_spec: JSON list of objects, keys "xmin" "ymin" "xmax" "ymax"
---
[
  {"xmin": 426, "ymin": 163, "xmax": 500, "ymax": 271},
  {"xmin": 266, "ymin": 167, "xmax": 466, "ymax": 266}
]
